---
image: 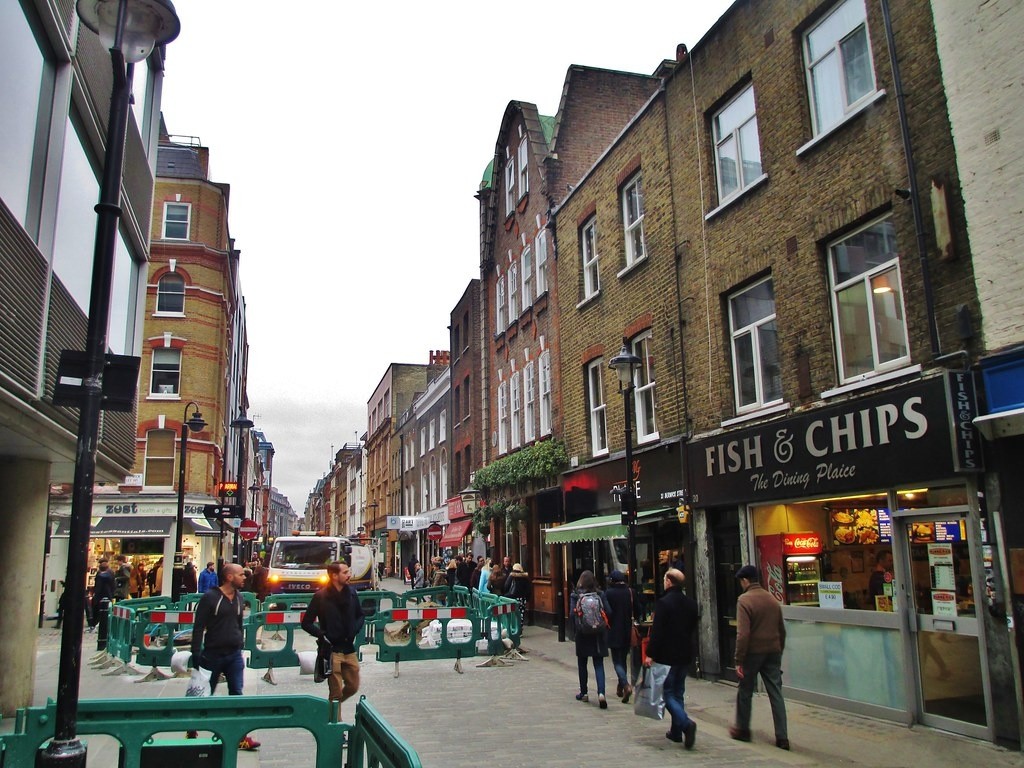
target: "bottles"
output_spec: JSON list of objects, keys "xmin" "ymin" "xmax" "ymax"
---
[{"xmin": 786, "ymin": 561, "xmax": 819, "ymax": 602}]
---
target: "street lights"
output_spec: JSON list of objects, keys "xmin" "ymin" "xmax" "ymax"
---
[
  {"xmin": 33, "ymin": 0, "xmax": 180, "ymax": 768},
  {"xmin": 370, "ymin": 498, "xmax": 379, "ymax": 558},
  {"xmin": 609, "ymin": 335, "xmax": 645, "ymax": 687},
  {"xmin": 248, "ymin": 478, "xmax": 260, "ymax": 562},
  {"xmin": 172, "ymin": 398, "xmax": 209, "ymax": 604}
]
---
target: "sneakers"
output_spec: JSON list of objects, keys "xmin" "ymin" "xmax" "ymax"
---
[
  {"xmin": 185, "ymin": 731, "xmax": 198, "ymax": 739},
  {"xmin": 237, "ymin": 736, "xmax": 260, "ymax": 750}
]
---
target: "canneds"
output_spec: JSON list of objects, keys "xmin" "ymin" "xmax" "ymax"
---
[
  {"xmin": 799, "ymin": 592, "xmax": 817, "ymax": 602},
  {"xmin": 788, "ymin": 562, "xmax": 801, "ymax": 581}
]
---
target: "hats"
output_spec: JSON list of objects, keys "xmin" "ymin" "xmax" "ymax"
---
[
  {"xmin": 609, "ymin": 571, "xmax": 624, "ymax": 581},
  {"xmin": 734, "ymin": 566, "xmax": 757, "ymax": 578}
]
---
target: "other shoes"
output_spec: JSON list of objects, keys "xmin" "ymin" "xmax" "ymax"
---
[
  {"xmin": 776, "ymin": 738, "xmax": 789, "ymax": 750},
  {"xmin": 576, "ymin": 693, "xmax": 588, "ymax": 701},
  {"xmin": 682, "ymin": 721, "xmax": 696, "ymax": 748},
  {"xmin": 342, "ymin": 734, "xmax": 348, "ymax": 749},
  {"xmin": 597, "ymin": 693, "xmax": 608, "ymax": 709},
  {"xmin": 729, "ymin": 726, "xmax": 750, "ymax": 741},
  {"xmin": 617, "ymin": 685, "xmax": 623, "ymax": 697},
  {"xmin": 665, "ymin": 730, "xmax": 682, "ymax": 742},
  {"xmin": 622, "ymin": 685, "xmax": 632, "ymax": 703}
]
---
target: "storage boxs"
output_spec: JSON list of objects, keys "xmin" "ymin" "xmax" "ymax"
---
[
  {"xmin": 875, "ymin": 595, "xmax": 894, "ymax": 612},
  {"xmin": 883, "ymin": 584, "xmax": 894, "ymax": 597}
]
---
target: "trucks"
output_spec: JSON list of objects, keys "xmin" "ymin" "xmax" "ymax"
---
[{"xmin": 265, "ymin": 529, "xmax": 380, "ymax": 610}]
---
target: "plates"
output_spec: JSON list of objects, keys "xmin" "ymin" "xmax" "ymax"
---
[{"xmin": 833, "ymin": 512, "xmax": 879, "ymax": 544}]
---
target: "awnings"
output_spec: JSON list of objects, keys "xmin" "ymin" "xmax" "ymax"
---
[
  {"xmin": 545, "ymin": 509, "xmax": 677, "ymax": 544},
  {"xmin": 188, "ymin": 518, "xmax": 228, "ymax": 537},
  {"xmin": 51, "ymin": 518, "xmax": 173, "ymax": 539},
  {"xmin": 439, "ymin": 520, "xmax": 475, "ymax": 547}
]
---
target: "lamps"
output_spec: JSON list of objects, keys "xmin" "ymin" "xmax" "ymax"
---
[{"xmin": 872, "ymin": 272, "xmax": 890, "ymax": 293}]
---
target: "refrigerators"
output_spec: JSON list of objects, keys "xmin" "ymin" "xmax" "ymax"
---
[{"xmin": 755, "ymin": 531, "xmax": 823, "ymax": 607}]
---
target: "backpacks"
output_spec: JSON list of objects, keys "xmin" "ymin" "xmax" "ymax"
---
[{"xmin": 575, "ymin": 589, "xmax": 610, "ymax": 634}]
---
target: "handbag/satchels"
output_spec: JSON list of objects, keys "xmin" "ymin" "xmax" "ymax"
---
[
  {"xmin": 314, "ymin": 636, "xmax": 333, "ymax": 684},
  {"xmin": 642, "ymin": 627, "xmax": 652, "ymax": 665},
  {"xmin": 631, "ymin": 627, "xmax": 640, "ymax": 648},
  {"xmin": 633, "ymin": 662, "xmax": 671, "ymax": 720},
  {"xmin": 185, "ymin": 666, "xmax": 212, "ymax": 697}
]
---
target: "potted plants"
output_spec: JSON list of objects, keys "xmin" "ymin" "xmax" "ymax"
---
[{"xmin": 473, "ymin": 493, "xmax": 527, "ymax": 536}]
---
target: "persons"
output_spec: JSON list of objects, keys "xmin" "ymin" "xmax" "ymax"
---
[
  {"xmin": 407, "ymin": 553, "xmax": 530, "ymax": 638},
  {"xmin": 643, "ymin": 569, "xmax": 700, "ymax": 749},
  {"xmin": 185, "ymin": 563, "xmax": 261, "ymax": 751},
  {"xmin": 729, "ymin": 565, "xmax": 791, "ymax": 751},
  {"xmin": 184, "ymin": 551, "xmax": 269, "ymax": 608},
  {"xmin": 870, "ymin": 550, "xmax": 893, "ymax": 611},
  {"xmin": 377, "ymin": 563, "xmax": 386, "ymax": 582},
  {"xmin": 569, "ymin": 571, "xmax": 610, "ymax": 709},
  {"xmin": 603, "ymin": 571, "xmax": 635, "ymax": 705},
  {"xmin": 300, "ymin": 561, "xmax": 365, "ymax": 747},
  {"xmin": 51, "ymin": 556, "xmax": 166, "ymax": 632}
]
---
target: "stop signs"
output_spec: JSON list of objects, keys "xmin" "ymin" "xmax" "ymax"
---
[
  {"xmin": 239, "ymin": 518, "xmax": 258, "ymax": 540},
  {"xmin": 428, "ymin": 524, "xmax": 442, "ymax": 541}
]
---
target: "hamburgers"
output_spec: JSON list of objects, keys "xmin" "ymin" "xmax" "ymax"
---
[{"xmin": 916, "ymin": 526, "xmax": 931, "ymax": 541}]
